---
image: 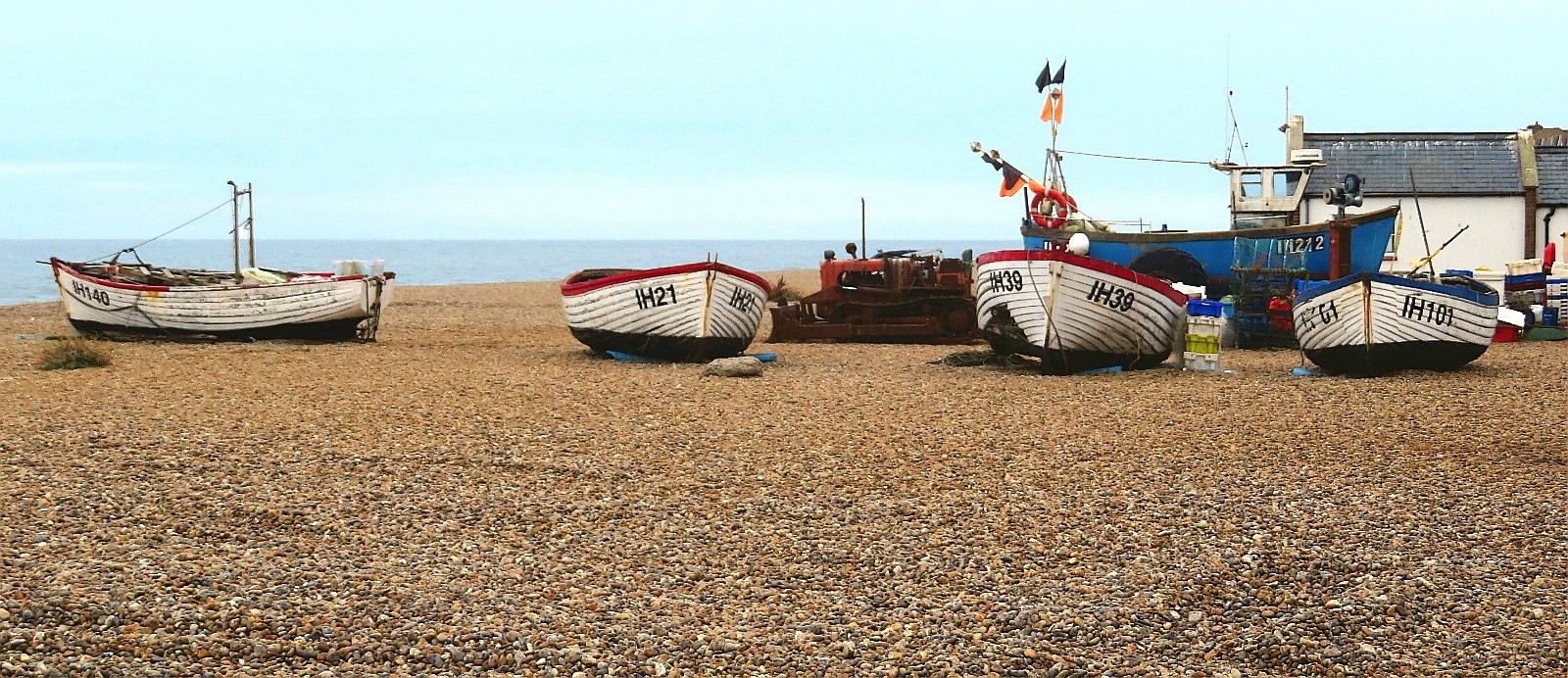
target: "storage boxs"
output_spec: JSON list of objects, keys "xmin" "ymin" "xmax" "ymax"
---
[
  {"xmin": 1183, "ymin": 300, "xmax": 1234, "ymax": 371},
  {"xmin": 1446, "ymin": 258, "xmax": 1568, "ymax": 342}
]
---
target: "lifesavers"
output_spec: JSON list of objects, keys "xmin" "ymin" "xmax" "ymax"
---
[
  {"xmin": 1513, "ymin": 307, "xmax": 1534, "ymax": 331},
  {"xmin": 1062, "ymin": 192, "xmax": 1077, "ymax": 214},
  {"xmin": 1032, "ymin": 190, "xmax": 1067, "ymax": 229}
]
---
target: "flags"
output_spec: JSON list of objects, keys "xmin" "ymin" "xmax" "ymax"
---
[
  {"xmin": 999, "ymin": 161, "xmax": 1026, "ymax": 197},
  {"xmin": 1036, "ymin": 60, "xmax": 1066, "ymax": 123}
]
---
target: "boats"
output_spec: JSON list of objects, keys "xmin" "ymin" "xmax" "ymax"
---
[
  {"xmin": 762, "ymin": 196, "xmax": 987, "ymax": 346},
  {"xmin": 969, "ymin": 84, "xmax": 1403, "ymax": 319},
  {"xmin": 559, "ymin": 251, "xmax": 774, "ymax": 364},
  {"xmin": 33, "ymin": 179, "xmax": 397, "ymax": 346},
  {"xmin": 972, "ymin": 232, "xmax": 1189, "ymax": 377},
  {"xmin": 1291, "ymin": 270, "xmax": 1501, "ymax": 380}
]
